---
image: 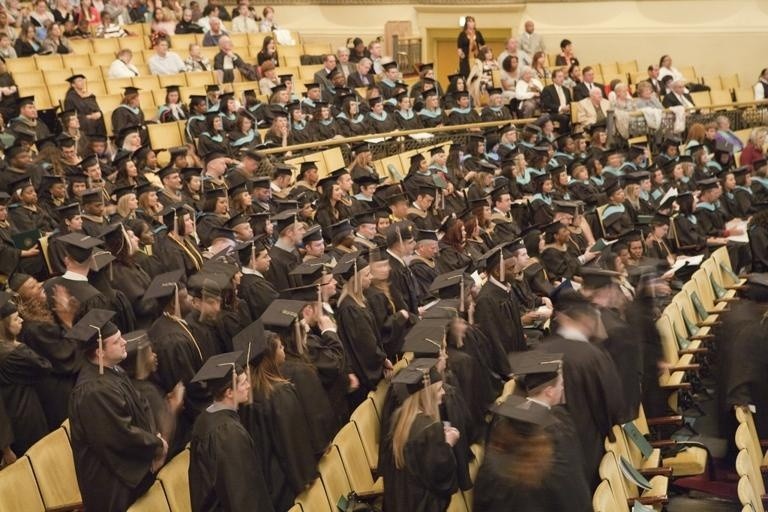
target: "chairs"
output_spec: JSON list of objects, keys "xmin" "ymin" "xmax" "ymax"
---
[{"xmin": 0, "ymin": 23, "xmax": 767, "ymax": 512}]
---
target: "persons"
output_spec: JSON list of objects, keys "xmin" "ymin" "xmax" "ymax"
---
[{"xmin": 0, "ymin": 0, "xmax": 768, "ymax": 512}]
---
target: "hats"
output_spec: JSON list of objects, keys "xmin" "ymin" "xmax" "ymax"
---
[{"xmin": 1, "ymin": 59, "xmax": 767, "ymax": 425}]
